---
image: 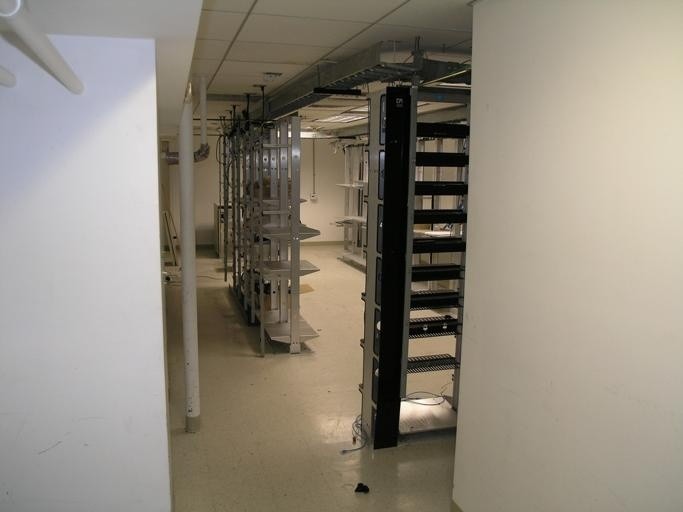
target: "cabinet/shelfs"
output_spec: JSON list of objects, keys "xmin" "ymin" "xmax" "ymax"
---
[{"xmin": 214, "ymin": 37, "xmax": 472, "ymax": 450}]
